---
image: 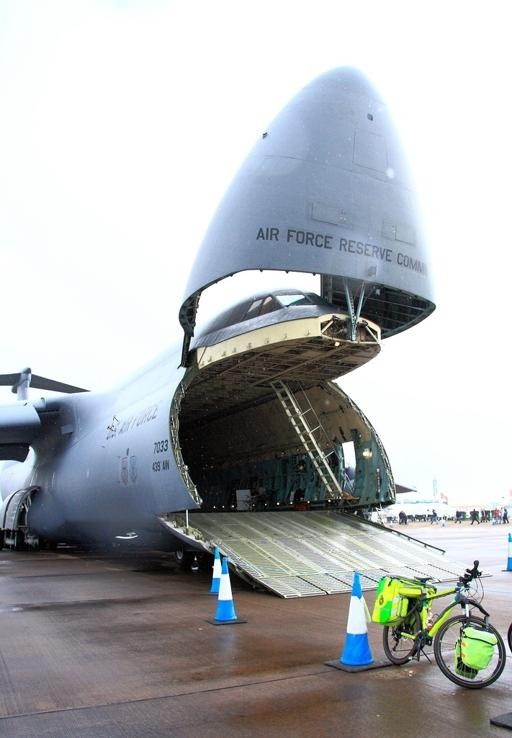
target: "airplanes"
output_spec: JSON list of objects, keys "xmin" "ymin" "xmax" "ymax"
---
[
  {"xmin": 1, "ymin": 65, "xmax": 469, "ymax": 602},
  {"xmin": 373, "ymin": 485, "xmax": 511, "ymax": 523}
]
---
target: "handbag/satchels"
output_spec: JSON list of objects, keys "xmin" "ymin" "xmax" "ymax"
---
[
  {"xmin": 454, "ymin": 627, "xmax": 498, "ymax": 680},
  {"xmin": 372, "ymin": 575, "xmax": 432, "ymax": 629}
]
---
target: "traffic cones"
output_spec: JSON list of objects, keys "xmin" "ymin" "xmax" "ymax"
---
[
  {"xmin": 209, "ymin": 543, "xmax": 234, "ymax": 594},
  {"xmin": 325, "ymin": 569, "xmax": 395, "ymax": 675},
  {"xmin": 201, "ymin": 554, "xmax": 251, "ymax": 626},
  {"xmin": 501, "ymin": 533, "xmax": 512, "ymax": 573}
]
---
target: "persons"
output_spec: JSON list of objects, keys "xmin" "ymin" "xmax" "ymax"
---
[{"xmin": 398, "ymin": 505, "xmax": 510, "ymax": 526}]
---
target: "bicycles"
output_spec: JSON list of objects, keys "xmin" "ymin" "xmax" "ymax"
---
[{"xmin": 370, "ymin": 556, "xmax": 508, "ymax": 694}]
